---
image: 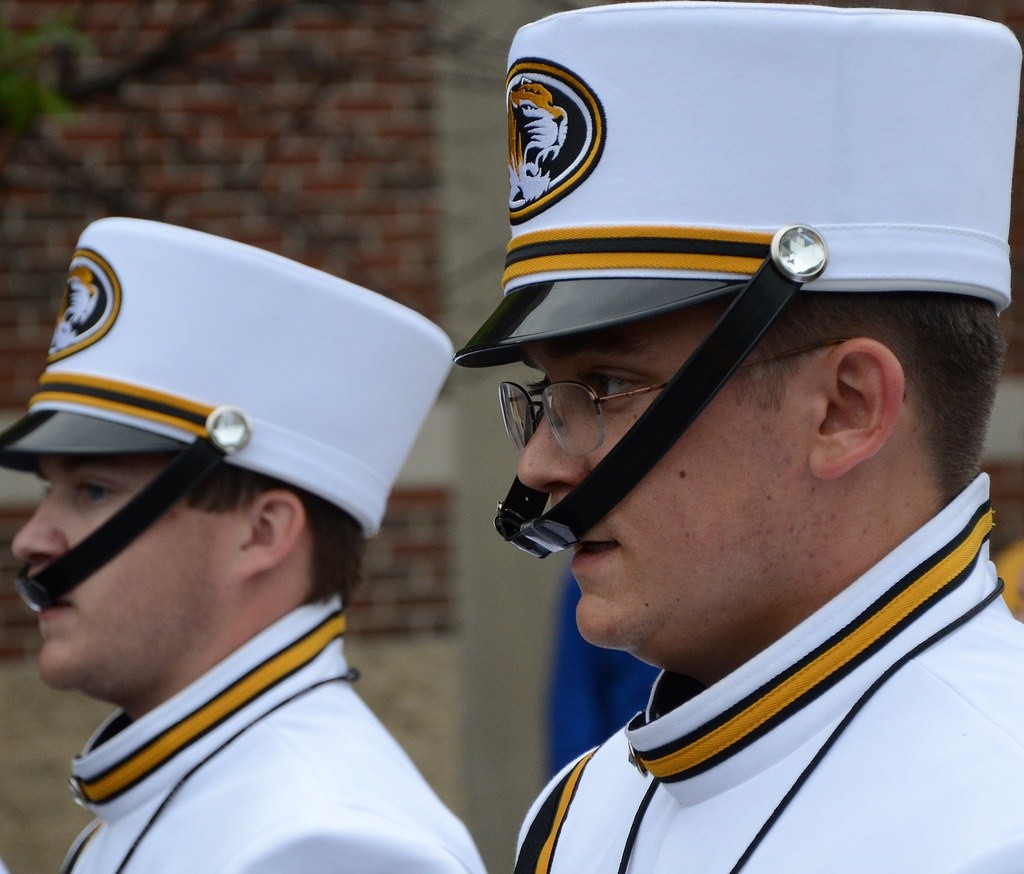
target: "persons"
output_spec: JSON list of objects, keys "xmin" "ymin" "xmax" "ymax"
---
[
  {"xmin": 508, "ymin": 89, "xmax": 1023, "ymax": 874},
  {"xmin": 10, "ymin": 315, "xmax": 489, "ymax": 874}
]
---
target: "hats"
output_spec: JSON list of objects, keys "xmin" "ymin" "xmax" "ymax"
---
[
  {"xmin": 0, "ymin": 217, "xmax": 455, "ymax": 614},
  {"xmin": 456, "ymin": 0, "xmax": 1022, "ymax": 565}
]
